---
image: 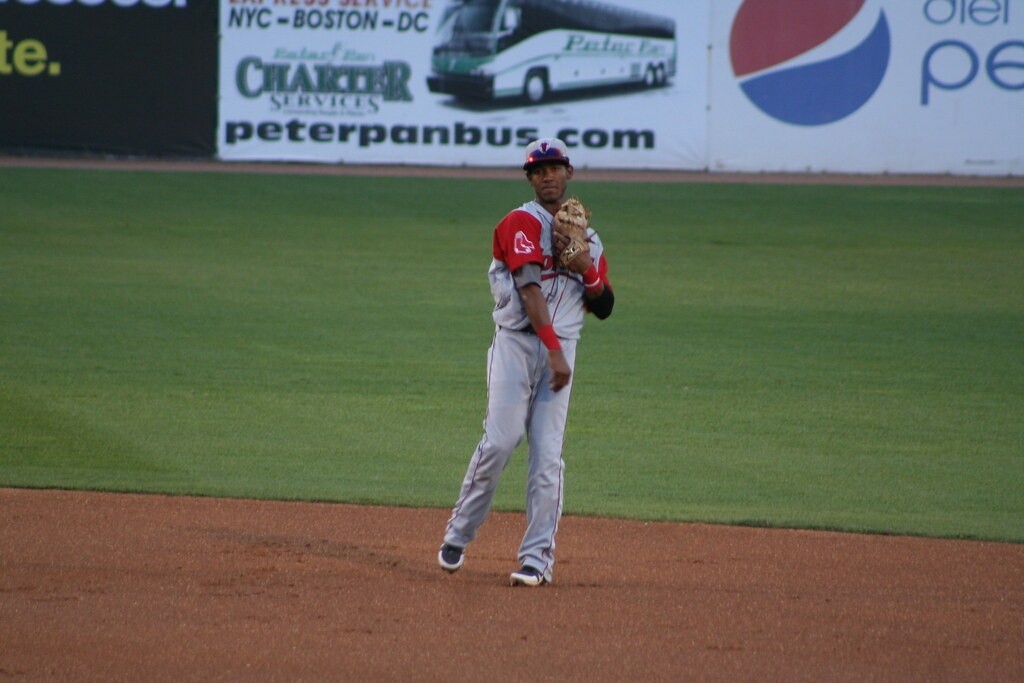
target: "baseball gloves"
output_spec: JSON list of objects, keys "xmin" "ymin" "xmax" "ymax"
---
[{"xmin": 551, "ymin": 197, "xmax": 593, "ymax": 270}]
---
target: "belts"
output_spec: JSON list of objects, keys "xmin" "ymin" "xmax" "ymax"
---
[{"xmin": 520, "ymin": 325, "xmax": 537, "ymax": 335}]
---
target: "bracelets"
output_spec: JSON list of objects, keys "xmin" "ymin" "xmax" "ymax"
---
[
  {"xmin": 537, "ymin": 324, "xmax": 562, "ymax": 352},
  {"xmin": 581, "ymin": 263, "xmax": 602, "ymax": 292}
]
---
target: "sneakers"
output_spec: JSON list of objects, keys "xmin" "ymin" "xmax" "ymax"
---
[
  {"xmin": 509, "ymin": 565, "xmax": 544, "ymax": 586},
  {"xmin": 437, "ymin": 542, "xmax": 465, "ymax": 570}
]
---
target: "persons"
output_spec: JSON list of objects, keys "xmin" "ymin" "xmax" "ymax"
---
[{"xmin": 438, "ymin": 136, "xmax": 615, "ymax": 587}]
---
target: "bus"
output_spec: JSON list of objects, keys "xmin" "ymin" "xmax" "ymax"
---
[{"xmin": 426, "ymin": 0, "xmax": 677, "ymax": 104}]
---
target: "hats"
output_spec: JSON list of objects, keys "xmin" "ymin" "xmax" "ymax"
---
[{"xmin": 522, "ymin": 137, "xmax": 569, "ymax": 170}]
